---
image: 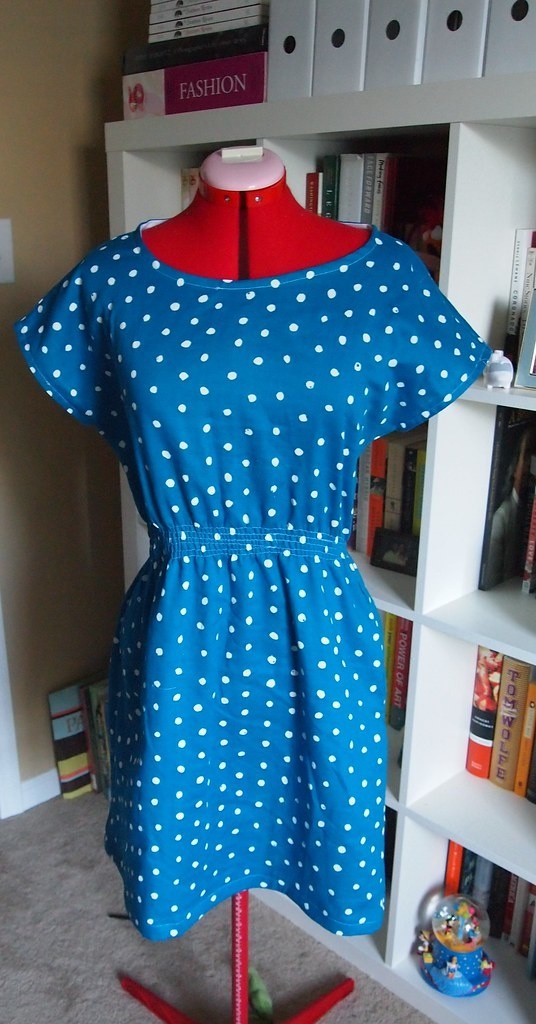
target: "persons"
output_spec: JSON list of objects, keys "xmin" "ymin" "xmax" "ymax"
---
[
  {"xmin": 475, "ymin": 650, "xmax": 498, "ymax": 712},
  {"xmin": 16, "ymin": 147, "xmax": 494, "ymax": 943},
  {"xmin": 488, "ymin": 430, "xmax": 533, "ymax": 586}
]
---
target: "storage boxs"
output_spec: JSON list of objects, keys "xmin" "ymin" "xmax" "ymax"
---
[{"xmin": 122, "ymin": 0, "xmax": 536, "ymax": 120}]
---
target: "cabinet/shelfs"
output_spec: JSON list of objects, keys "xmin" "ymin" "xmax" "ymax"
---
[{"xmin": 105, "ymin": 73, "xmax": 536, "ymax": 1024}]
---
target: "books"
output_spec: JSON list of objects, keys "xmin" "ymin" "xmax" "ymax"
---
[
  {"xmin": 504, "ymin": 229, "xmax": 536, "ymax": 370},
  {"xmin": 384, "ymin": 613, "xmax": 413, "ymax": 767},
  {"xmin": 479, "ymin": 406, "xmax": 536, "ymax": 593},
  {"xmin": 182, "ymin": 167, "xmax": 200, "ymax": 213},
  {"xmin": 348, "ymin": 430, "xmax": 426, "ymax": 557},
  {"xmin": 48, "ymin": 671, "xmax": 112, "ymax": 800},
  {"xmin": 306, "ymin": 152, "xmax": 447, "ymax": 287},
  {"xmin": 443, "ymin": 839, "xmax": 536, "ymax": 957},
  {"xmin": 466, "ymin": 645, "xmax": 536, "ymax": 804}
]
